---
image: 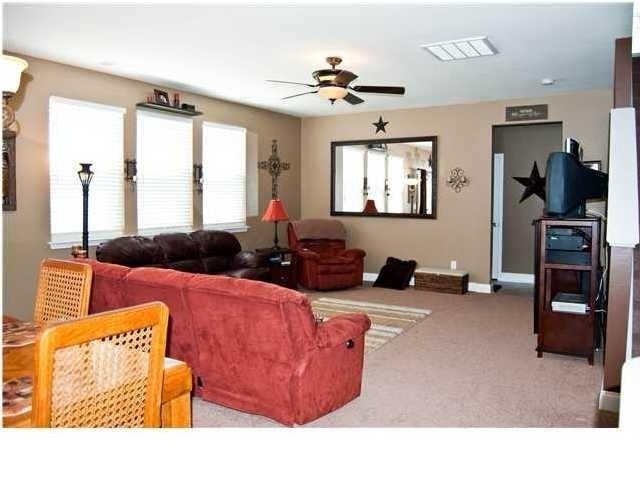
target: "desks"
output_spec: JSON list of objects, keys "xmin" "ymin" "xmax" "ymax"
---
[{"xmin": 4, "ymin": 314, "xmax": 196, "ymax": 428}]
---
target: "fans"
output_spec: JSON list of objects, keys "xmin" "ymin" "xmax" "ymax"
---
[{"xmin": 261, "ymin": 55, "xmax": 407, "ymax": 107}]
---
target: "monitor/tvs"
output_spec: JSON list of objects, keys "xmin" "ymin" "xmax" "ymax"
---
[{"xmin": 546, "ymin": 152, "xmax": 608, "ymax": 218}]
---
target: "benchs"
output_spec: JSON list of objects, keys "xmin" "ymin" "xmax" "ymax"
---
[{"xmin": 414, "ymin": 267, "xmax": 469, "ymax": 296}]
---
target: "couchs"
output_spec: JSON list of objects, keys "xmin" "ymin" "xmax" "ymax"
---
[
  {"xmin": 69, "ymin": 231, "xmax": 371, "ymax": 428},
  {"xmin": 262, "ymin": 198, "xmax": 291, "ymax": 252}
]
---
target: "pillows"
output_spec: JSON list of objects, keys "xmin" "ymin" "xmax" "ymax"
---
[{"xmin": 373, "ymin": 257, "xmax": 417, "ymax": 290}]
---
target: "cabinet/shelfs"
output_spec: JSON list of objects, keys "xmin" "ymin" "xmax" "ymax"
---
[{"xmin": 530, "ymin": 217, "xmax": 601, "ymax": 367}]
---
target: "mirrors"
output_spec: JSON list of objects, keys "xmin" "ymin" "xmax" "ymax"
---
[{"xmin": 329, "ymin": 136, "xmax": 438, "ymax": 220}]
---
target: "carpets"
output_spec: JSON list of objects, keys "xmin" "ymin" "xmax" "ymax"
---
[{"xmin": 303, "ymin": 289, "xmax": 433, "ymax": 358}]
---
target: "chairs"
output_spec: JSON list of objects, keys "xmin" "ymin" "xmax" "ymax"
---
[
  {"xmin": 288, "ymin": 219, "xmax": 366, "ymax": 293},
  {"xmin": 33, "ymin": 259, "xmax": 171, "ymax": 428}
]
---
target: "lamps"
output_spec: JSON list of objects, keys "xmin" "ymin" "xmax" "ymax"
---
[{"xmin": 318, "ymin": 85, "xmax": 349, "ymax": 106}]
